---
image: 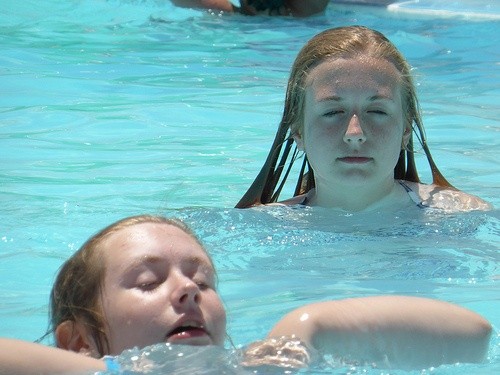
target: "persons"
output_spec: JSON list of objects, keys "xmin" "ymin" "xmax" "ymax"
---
[
  {"xmin": 0, "ymin": 216, "xmax": 494, "ymax": 375},
  {"xmin": 234, "ymin": 25, "xmax": 493, "ymax": 235},
  {"xmin": 169, "ymin": 0, "xmax": 330, "ymax": 19}
]
